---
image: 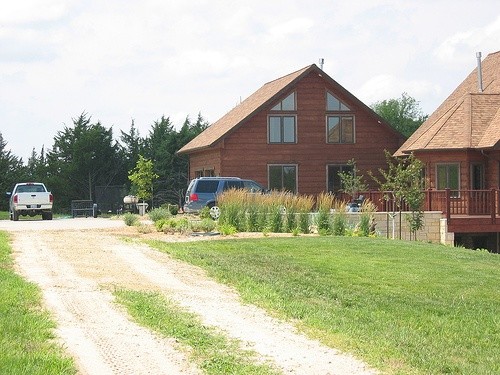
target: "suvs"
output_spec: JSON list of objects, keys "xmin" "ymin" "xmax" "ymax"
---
[{"xmin": 183, "ymin": 177, "xmax": 271, "ymax": 219}]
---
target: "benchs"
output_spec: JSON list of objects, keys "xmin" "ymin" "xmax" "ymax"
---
[{"xmin": 70, "ymin": 198, "xmax": 99, "ymax": 219}]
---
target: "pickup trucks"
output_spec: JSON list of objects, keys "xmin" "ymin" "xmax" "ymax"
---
[{"xmin": 10, "ymin": 183, "xmax": 54, "ymax": 220}]
